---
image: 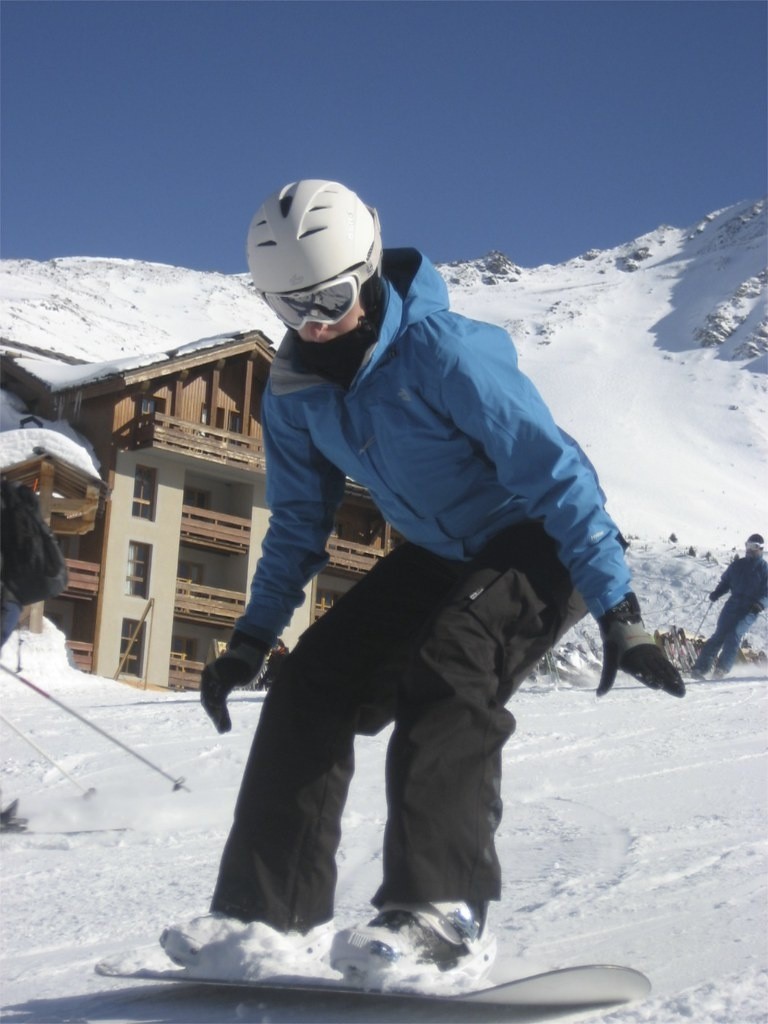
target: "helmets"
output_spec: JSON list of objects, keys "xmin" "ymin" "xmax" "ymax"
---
[
  {"xmin": 747, "ymin": 534, "xmax": 764, "ymax": 547},
  {"xmin": 245, "ymin": 177, "xmax": 382, "ymax": 294}
]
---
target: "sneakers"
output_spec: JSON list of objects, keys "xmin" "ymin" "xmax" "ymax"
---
[
  {"xmin": 156, "ymin": 902, "xmax": 327, "ymax": 965},
  {"xmin": 333, "ymin": 909, "xmax": 476, "ymax": 982}
]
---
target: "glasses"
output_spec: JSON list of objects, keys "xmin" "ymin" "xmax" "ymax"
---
[
  {"xmin": 259, "ymin": 277, "xmax": 358, "ymax": 331},
  {"xmin": 745, "ymin": 542, "xmax": 760, "ymax": 550}
]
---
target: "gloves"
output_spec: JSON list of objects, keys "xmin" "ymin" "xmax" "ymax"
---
[
  {"xmin": 595, "ymin": 592, "xmax": 686, "ymax": 698},
  {"xmin": 198, "ymin": 629, "xmax": 269, "ymax": 731}
]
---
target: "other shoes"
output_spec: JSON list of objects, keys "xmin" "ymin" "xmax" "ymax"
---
[
  {"xmin": 690, "ymin": 666, "xmax": 705, "ymax": 680},
  {"xmin": 714, "ymin": 666, "xmax": 726, "ymax": 679}
]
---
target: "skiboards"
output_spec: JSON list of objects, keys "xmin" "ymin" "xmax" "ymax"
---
[{"xmin": 0, "ymin": 784, "xmax": 96, "ymax": 831}]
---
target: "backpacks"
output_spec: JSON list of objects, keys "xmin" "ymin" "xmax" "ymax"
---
[{"xmin": 2, "ymin": 480, "xmax": 71, "ymax": 604}]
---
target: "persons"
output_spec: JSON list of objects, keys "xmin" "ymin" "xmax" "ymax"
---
[
  {"xmin": 691, "ymin": 534, "xmax": 768, "ymax": 681},
  {"xmin": 256, "ymin": 637, "xmax": 290, "ymax": 692},
  {"xmin": 161, "ymin": 178, "xmax": 685, "ymax": 997}
]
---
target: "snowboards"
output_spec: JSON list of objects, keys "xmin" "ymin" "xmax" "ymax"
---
[{"xmin": 96, "ymin": 963, "xmax": 658, "ymax": 1008}]
